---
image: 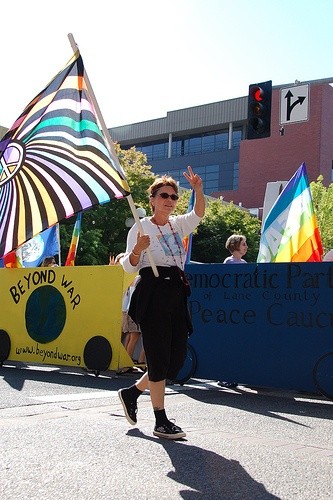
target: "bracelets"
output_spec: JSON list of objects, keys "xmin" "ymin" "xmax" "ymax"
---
[{"xmin": 131, "ymin": 249, "xmax": 140, "ymax": 256}]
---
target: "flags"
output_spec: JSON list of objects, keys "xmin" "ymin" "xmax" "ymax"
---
[
  {"xmin": 0, "ymin": 47, "xmax": 131, "ymax": 258},
  {"xmin": 0, "ymin": 224, "xmax": 61, "ymax": 268},
  {"xmin": 258, "ymin": 162, "xmax": 323, "ymax": 263},
  {"xmin": 65, "ymin": 212, "xmax": 81, "ymax": 266}
]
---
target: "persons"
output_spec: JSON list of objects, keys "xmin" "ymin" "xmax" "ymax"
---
[
  {"xmin": 224, "ymin": 235, "xmax": 249, "ymax": 264},
  {"xmin": 41, "ymin": 256, "xmax": 56, "ymax": 267},
  {"xmin": 109, "ymin": 253, "xmax": 147, "ymax": 374},
  {"xmin": 118, "ymin": 164, "xmax": 205, "ymax": 439}
]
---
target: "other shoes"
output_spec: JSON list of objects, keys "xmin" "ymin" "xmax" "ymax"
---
[
  {"xmin": 153, "ymin": 418, "xmax": 186, "ymax": 438},
  {"xmin": 118, "ymin": 387, "xmax": 139, "ymax": 425}
]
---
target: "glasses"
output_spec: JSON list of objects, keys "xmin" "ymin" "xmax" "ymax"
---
[{"xmin": 155, "ymin": 193, "xmax": 179, "ymax": 200}]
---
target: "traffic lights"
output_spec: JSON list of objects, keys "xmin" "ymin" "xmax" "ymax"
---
[{"xmin": 243, "ymin": 79, "xmax": 272, "ymax": 140}]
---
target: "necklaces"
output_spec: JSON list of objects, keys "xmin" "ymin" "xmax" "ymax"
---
[{"xmin": 151, "ymin": 214, "xmax": 190, "ymax": 286}]
